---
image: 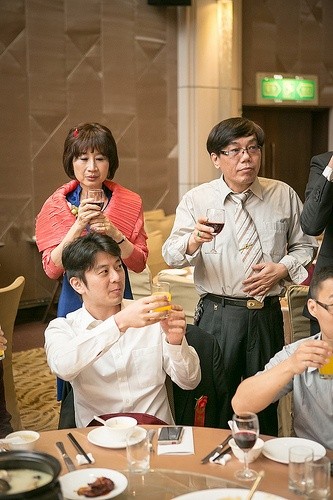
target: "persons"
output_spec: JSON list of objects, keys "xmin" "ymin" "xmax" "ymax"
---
[
  {"xmin": 35, "ymin": 123, "xmax": 149, "ymax": 400},
  {"xmin": 0, "ymin": 326, "xmax": 13, "ymax": 440},
  {"xmin": 229, "ymin": 151, "xmax": 333, "ymax": 451},
  {"xmin": 161, "ymin": 118, "xmax": 319, "ymax": 439},
  {"xmin": 44, "ymin": 234, "xmax": 201, "ymax": 426}
]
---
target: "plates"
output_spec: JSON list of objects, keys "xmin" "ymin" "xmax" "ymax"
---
[
  {"xmin": 58, "ymin": 467, "xmax": 128, "ymax": 500},
  {"xmin": 87, "ymin": 426, "xmax": 148, "ymax": 448},
  {"xmin": 171, "ymin": 488, "xmax": 287, "ymax": 500},
  {"xmin": 261, "ymin": 437, "xmax": 326, "ymax": 464}
]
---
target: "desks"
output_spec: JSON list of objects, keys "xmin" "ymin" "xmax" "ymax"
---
[{"xmin": 150, "ymin": 265, "xmax": 200, "ymax": 325}]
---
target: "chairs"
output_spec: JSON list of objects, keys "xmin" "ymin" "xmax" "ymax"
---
[
  {"xmin": 0, "ymin": 276, "xmax": 24, "ymax": 433},
  {"xmin": 127, "ymin": 209, "xmax": 175, "ymax": 301}
]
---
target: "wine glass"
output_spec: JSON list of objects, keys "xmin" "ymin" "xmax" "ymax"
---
[
  {"xmin": 232, "ymin": 411, "xmax": 259, "ymax": 481},
  {"xmin": 204, "ymin": 208, "xmax": 225, "ymax": 254}
]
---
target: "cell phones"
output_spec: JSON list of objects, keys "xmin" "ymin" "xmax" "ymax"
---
[{"xmin": 158, "ymin": 426, "xmax": 183, "ymax": 445}]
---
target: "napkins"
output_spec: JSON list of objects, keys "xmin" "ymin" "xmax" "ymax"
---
[{"xmin": 158, "ymin": 426, "xmax": 195, "ymax": 456}]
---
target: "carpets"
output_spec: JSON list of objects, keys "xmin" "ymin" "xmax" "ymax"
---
[{"xmin": 10, "ymin": 347, "xmax": 61, "ymax": 432}]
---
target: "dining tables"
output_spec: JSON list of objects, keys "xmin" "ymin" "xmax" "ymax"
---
[{"xmin": 0, "ymin": 424, "xmax": 333, "ymax": 500}]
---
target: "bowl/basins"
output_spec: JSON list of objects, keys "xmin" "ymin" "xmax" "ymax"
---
[
  {"xmin": 104, "ymin": 416, "xmax": 137, "ymax": 441},
  {"xmin": 228, "ymin": 438, "xmax": 264, "ymax": 464},
  {"xmin": 0, "ymin": 450, "xmax": 62, "ymax": 500},
  {"xmin": 5, "ymin": 430, "xmax": 40, "ymax": 451}
]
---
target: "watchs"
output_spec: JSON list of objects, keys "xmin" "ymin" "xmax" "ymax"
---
[{"xmin": 117, "ymin": 236, "xmax": 127, "ymax": 245}]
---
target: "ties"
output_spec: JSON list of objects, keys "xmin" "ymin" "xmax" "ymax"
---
[{"xmin": 226, "ymin": 190, "xmax": 271, "ymax": 303}]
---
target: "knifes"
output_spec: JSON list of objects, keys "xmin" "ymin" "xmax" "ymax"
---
[
  {"xmin": 55, "ymin": 441, "xmax": 76, "ymax": 472},
  {"xmin": 200, "ymin": 433, "xmax": 232, "ymax": 464}
]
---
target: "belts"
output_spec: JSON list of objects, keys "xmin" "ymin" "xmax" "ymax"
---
[{"xmin": 204, "ymin": 293, "xmax": 279, "ymax": 309}]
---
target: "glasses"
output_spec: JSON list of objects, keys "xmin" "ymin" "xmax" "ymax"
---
[
  {"xmin": 219, "ymin": 144, "xmax": 262, "ymax": 159},
  {"xmin": 313, "ymin": 299, "xmax": 333, "ymax": 314}
]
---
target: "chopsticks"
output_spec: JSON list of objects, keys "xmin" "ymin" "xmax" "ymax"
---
[{"xmin": 68, "ymin": 432, "xmax": 92, "ymax": 463}]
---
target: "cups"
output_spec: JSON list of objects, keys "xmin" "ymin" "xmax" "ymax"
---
[
  {"xmin": 125, "ymin": 429, "xmax": 151, "ymax": 474},
  {"xmin": 151, "ymin": 282, "xmax": 171, "ymax": 318},
  {"xmin": 318, "ymin": 340, "xmax": 333, "ymax": 380},
  {"xmin": 86, "ymin": 189, "xmax": 105, "ymax": 211},
  {"xmin": 304, "ymin": 454, "xmax": 331, "ymax": 500},
  {"xmin": 288, "ymin": 446, "xmax": 315, "ymax": 495}
]
